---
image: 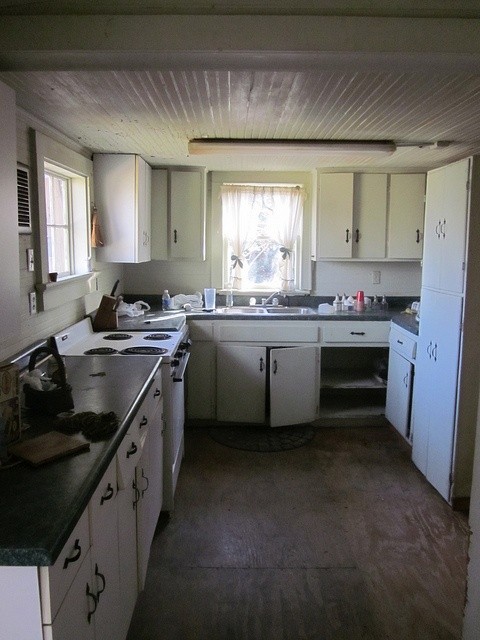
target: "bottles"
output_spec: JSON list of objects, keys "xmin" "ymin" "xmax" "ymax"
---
[
  {"xmin": 333, "ymin": 293, "xmax": 342, "ymax": 310},
  {"xmin": 162, "ymin": 290, "xmax": 170, "ymax": 311},
  {"xmin": 381, "ymin": 295, "xmax": 388, "ymax": 310},
  {"xmin": 225, "ymin": 284, "xmax": 233, "ymax": 306},
  {"xmin": 342, "ymin": 293, "xmax": 347, "ymax": 311},
  {"xmin": 372, "ymin": 296, "xmax": 380, "ymax": 310},
  {"xmin": 356, "ymin": 291, "xmax": 364, "ymax": 312}
]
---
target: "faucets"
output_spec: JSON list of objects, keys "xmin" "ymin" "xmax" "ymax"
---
[{"xmin": 262, "ymin": 287, "xmax": 282, "ymax": 308}]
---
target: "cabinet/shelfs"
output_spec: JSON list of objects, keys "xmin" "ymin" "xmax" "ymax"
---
[
  {"xmin": 410, "ymin": 287, "xmax": 478, "ymax": 513},
  {"xmin": 0, "ymin": 454, "xmax": 124, "ymax": 640},
  {"xmin": 319, "ymin": 314, "xmax": 390, "ymax": 426},
  {"xmin": 421, "ymin": 154, "xmax": 479, "ymax": 297},
  {"xmin": 385, "ymin": 319, "xmax": 418, "ymax": 445},
  {"xmin": 91, "ymin": 150, "xmax": 151, "ymax": 265},
  {"xmin": 215, "ymin": 317, "xmax": 320, "ymax": 429},
  {"xmin": 115, "ymin": 355, "xmax": 165, "ymax": 636},
  {"xmin": 309, "ymin": 166, "xmax": 388, "ymax": 262},
  {"xmin": 150, "ymin": 165, "xmax": 208, "ymax": 262},
  {"xmin": 385, "ymin": 166, "xmax": 427, "ymax": 262}
]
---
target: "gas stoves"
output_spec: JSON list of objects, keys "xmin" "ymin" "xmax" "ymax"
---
[{"xmin": 50, "ymin": 317, "xmax": 190, "ymax": 382}]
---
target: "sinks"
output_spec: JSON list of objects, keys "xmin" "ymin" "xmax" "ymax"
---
[
  {"xmin": 217, "ymin": 306, "xmax": 268, "ymax": 315},
  {"xmin": 265, "ymin": 306, "xmax": 317, "ymax": 317}
]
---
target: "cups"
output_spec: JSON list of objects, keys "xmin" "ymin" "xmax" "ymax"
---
[{"xmin": 204, "ymin": 288, "xmax": 216, "ymax": 309}]
